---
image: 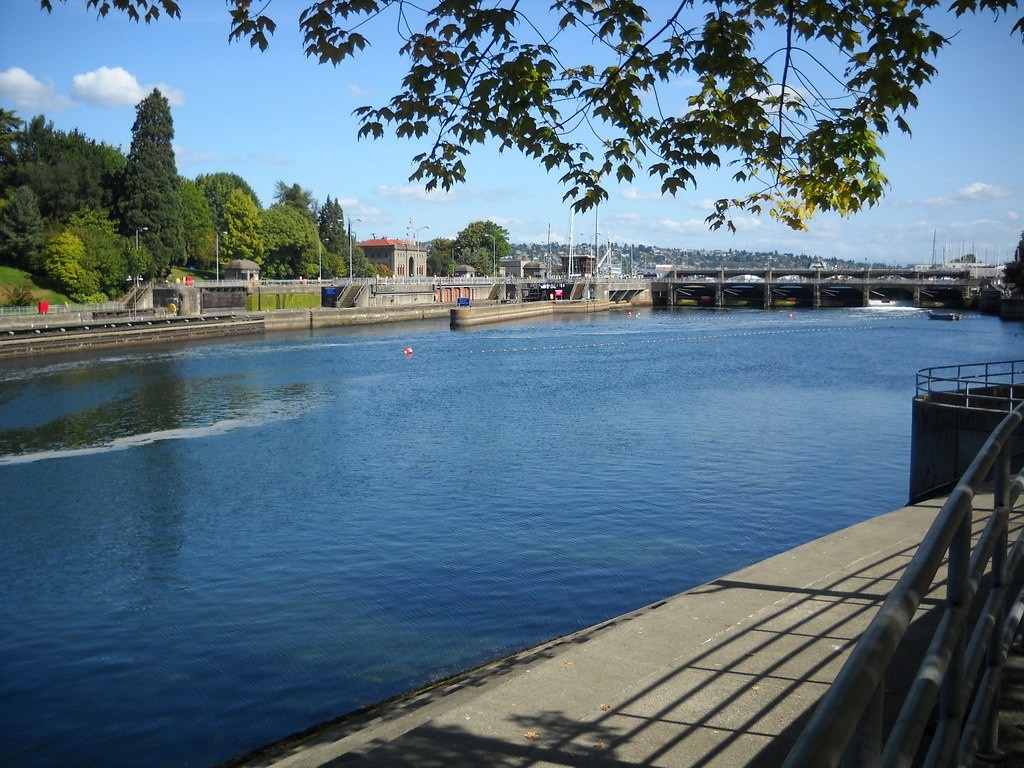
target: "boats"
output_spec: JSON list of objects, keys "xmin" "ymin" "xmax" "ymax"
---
[{"xmin": 927, "ymin": 310, "xmax": 965, "ymax": 321}]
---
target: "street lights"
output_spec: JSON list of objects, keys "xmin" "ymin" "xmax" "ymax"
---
[
  {"xmin": 571, "ymin": 233, "xmax": 584, "ymax": 276},
  {"xmin": 216, "ymin": 231, "xmax": 228, "ymax": 283},
  {"xmin": 135, "ymin": 227, "xmax": 148, "ymax": 250},
  {"xmin": 417, "ymin": 225, "xmax": 430, "ymax": 277},
  {"xmin": 485, "ymin": 233, "xmax": 496, "ymax": 277},
  {"xmin": 350, "ymin": 219, "xmax": 361, "ymax": 281},
  {"xmin": 451, "ymin": 247, "xmax": 461, "ymax": 277},
  {"xmin": 318, "ymin": 238, "xmax": 330, "ymax": 280},
  {"xmin": 590, "ymin": 232, "xmax": 602, "ymax": 276}
]
